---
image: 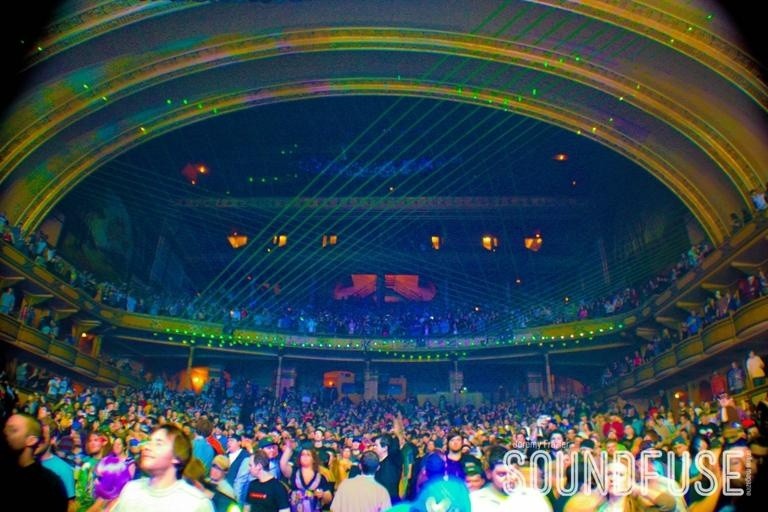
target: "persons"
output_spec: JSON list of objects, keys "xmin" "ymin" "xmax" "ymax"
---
[{"xmin": 0, "ymin": 189, "xmax": 768, "ymax": 512}]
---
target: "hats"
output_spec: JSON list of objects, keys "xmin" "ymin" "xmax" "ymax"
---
[
  {"xmin": 258, "ymin": 437, "xmax": 277, "ymax": 448},
  {"xmin": 670, "ymin": 434, "xmax": 687, "ymax": 446}
]
